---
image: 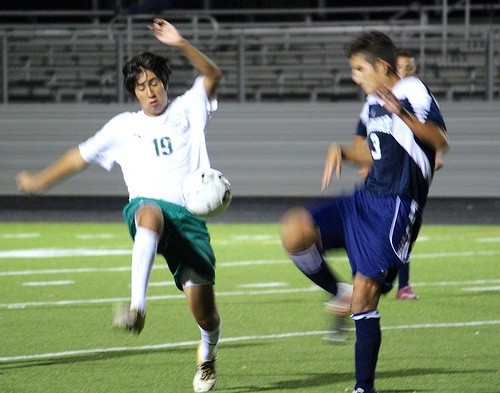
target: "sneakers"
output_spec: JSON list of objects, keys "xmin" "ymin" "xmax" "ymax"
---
[
  {"xmin": 396, "ymin": 286, "xmax": 417, "ymax": 300},
  {"xmin": 192, "ymin": 341, "xmax": 216, "ymax": 393},
  {"xmin": 113, "ymin": 308, "xmax": 146, "ymax": 337}
]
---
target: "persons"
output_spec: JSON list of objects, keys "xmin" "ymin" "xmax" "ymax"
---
[
  {"xmin": 15, "ymin": 18, "xmax": 239, "ymax": 393},
  {"xmin": 279, "ymin": 29, "xmax": 450, "ymax": 393}
]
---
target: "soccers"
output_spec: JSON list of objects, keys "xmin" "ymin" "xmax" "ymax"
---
[{"xmin": 182, "ymin": 168, "xmax": 232, "ymax": 216}]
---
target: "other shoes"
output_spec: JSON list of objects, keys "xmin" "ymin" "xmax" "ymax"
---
[{"xmin": 322, "ymin": 282, "xmax": 356, "ymax": 343}]
